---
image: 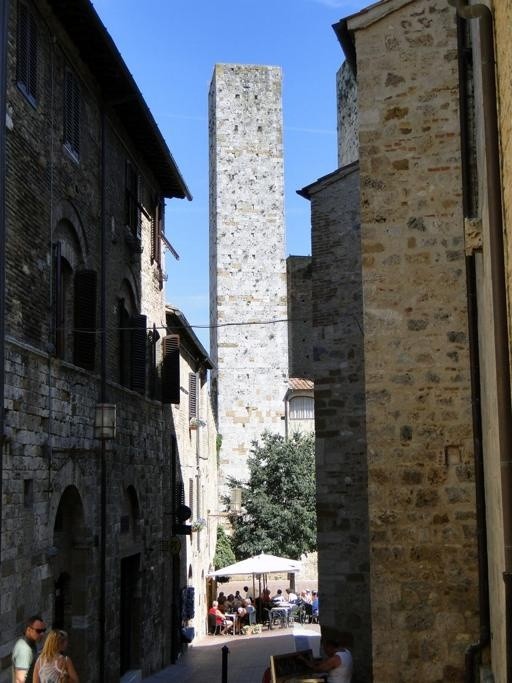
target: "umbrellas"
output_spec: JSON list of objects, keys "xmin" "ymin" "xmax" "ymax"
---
[{"xmin": 207, "ymin": 551, "xmax": 308, "ymax": 597}]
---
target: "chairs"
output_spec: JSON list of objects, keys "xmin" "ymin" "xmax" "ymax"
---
[{"xmin": 208, "ymin": 602, "xmax": 319, "ymax": 637}]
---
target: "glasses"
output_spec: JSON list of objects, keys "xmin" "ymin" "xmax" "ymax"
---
[{"xmin": 29, "ymin": 626, "xmax": 47, "ymax": 634}]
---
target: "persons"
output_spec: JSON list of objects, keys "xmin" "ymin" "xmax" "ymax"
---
[
  {"xmin": 10, "ymin": 615, "xmax": 47, "ymax": 683},
  {"xmin": 207, "ymin": 583, "xmax": 320, "ymax": 635},
  {"xmin": 30, "ymin": 628, "xmax": 82, "ymax": 683},
  {"xmin": 297, "ymin": 628, "xmax": 357, "ymax": 680}
]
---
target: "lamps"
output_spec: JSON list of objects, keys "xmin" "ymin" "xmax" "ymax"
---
[{"xmin": 93, "ymin": 401, "xmax": 119, "ymax": 441}]
